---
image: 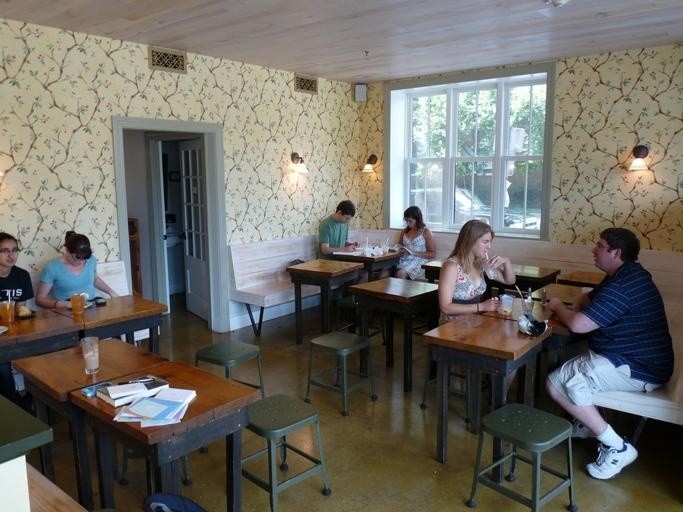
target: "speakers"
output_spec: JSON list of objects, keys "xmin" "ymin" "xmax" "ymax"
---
[{"xmin": 354, "ymin": 85, "xmax": 367, "ymax": 102}]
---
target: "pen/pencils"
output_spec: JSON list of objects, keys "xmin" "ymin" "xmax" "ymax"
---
[{"xmin": 118, "ymin": 379, "xmax": 154, "ymax": 385}]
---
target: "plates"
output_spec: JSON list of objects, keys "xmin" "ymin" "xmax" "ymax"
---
[{"xmin": 17, "ymin": 310, "xmax": 36, "ymax": 319}]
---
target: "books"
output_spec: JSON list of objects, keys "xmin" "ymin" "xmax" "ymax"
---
[
  {"xmin": 333, "ymin": 246, "xmax": 374, "ymax": 257},
  {"xmin": 392, "ymin": 243, "xmax": 413, "ymax": 256},
  {"xmin": 96, "ymin": 373, "xmax": 198, "ymax": 428}
]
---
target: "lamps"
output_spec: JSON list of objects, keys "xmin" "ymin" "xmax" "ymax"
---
[
  {"xmin": 362, "ymin": 154, "xmax": 380, "ymax": 175},
  {"xmin": 627, "ymin": 144, "xmax": 651, "ymax": 173}
]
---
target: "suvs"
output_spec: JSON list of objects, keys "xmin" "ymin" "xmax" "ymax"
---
[{"xmin": 411, "ymin": 185, "xmax": 518, "ymax": 226}]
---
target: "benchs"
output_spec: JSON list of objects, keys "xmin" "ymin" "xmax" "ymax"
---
[
  {"xmin": 26, "ymin": 260, "xmax": 160, "ymax": 343},
  {"xmin": 228, "ymin": 234, "xmax": 356, "ymax": 340}
]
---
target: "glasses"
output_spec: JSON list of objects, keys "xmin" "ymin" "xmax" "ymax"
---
[
  {"xmin": 0, "ymin": 247, "xmax": 21, "ymax": 255},
  {"xmin": 71, "ymin": 254, "xmax": 90, "ymax": 264}
]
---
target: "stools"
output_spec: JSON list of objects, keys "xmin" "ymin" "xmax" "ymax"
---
[
  {"xmin": 466, "ymin": 403, "xmax": 576, "ymax": 510},
  {"xmin": 241, "ymin": 393, "xmax": 331, "ymax": 510}
]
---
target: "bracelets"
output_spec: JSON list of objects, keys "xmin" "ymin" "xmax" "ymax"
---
[{"xmin": 475, "ymin": 303, "xmax": 480, "ymax": 314}]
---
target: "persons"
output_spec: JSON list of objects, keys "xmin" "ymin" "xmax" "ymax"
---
[
  {"xmin": 377, "ymin": 205, "xmax": 438, "ymax": 280},
  {"xmin": 34, "ymin": 230, "xmax": 120, "ymax": 311},
  {"xmin": 1, "ymin": 232, "xmax": 38, "ymax": 418},
  {"xmin": 317, "ymin": 199, "xmax": 370, "ymax": 285},
  {"xmin": 436, "ymin": 219, "xmax": 519, "ymax": 413},
  {"xmin": 542, "ymin": 226, "xmax": 675, "ymax": 482}
]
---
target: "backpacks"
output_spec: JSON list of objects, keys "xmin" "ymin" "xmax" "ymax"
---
[{"xmin": 144, "ymin": 492, "xmax": 207, "ymax": 512}]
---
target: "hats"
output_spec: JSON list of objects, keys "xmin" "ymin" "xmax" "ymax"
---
[{"xmin": 517, "ymin": 314, "xmax": 548, "ymax": 337}]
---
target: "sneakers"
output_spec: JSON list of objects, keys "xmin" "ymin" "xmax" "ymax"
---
[
  {"xmin": 583, "ymin": 435, "xmax": 638, "ymax": 480},
  {"xmin": 572, "ymin": 418, "xmax": 598, "ymax": 439}
]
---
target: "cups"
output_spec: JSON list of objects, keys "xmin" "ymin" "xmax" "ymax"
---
[
  {"xmin": 72, "ymin": 292, "xmax": 84, "ymax": 315},
  {"xmin": 499, "ymin": 295, "xmax": 513, "ymax": 317},
  {"xmin": 363, "ymin": 242, "xmax": 372, "ymax": 257},
  {"xmin": 522, "ymin": 299, "xmax": 534, "ymax": 314},
  {"xmin": 380, "ymin": 241, "xmax": 389, "ymax": 255},
  {"xmin": 0, "ymin": 300, "xmax": 16, "ymax": 323},
  {"xmin": 82, "ymin": 336, "xmax": 100, "ymax": 376}
]
